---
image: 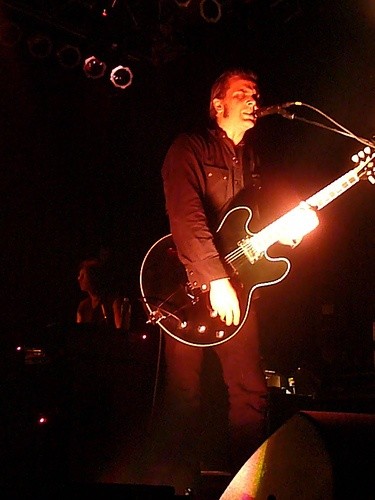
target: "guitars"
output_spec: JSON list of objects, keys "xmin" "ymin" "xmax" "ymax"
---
[{"xmin": 138, "ymin": 137, "xmax": 375, "ymax": 347}]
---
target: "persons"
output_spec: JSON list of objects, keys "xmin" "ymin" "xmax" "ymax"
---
[
  {"xmin": 75, "ymin": 253, "xmax": 132, "ymax": 331},
  {"xmin": 159, "ymin": 70, "xmax": 321, "ymax": 480}
]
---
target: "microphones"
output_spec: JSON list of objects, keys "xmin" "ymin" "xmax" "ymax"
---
[{"xmin": 251, "ymin": 101, "xmax": 302, "ymax": 119}]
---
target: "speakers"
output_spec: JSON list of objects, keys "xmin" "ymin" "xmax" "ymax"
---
[{"xmin": 219, "ymin": 411, "xmax": 375, "ymax": 500}]
